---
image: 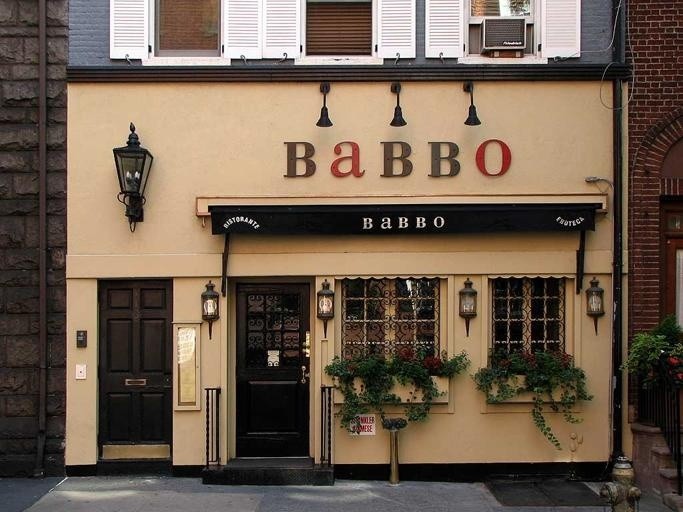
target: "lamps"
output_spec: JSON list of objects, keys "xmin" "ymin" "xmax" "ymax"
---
[
  {"xmin": 316, "ymin": 82, "xmax": 333, "ymax": 127},
  {"xmin": 390, "ymin": 82, "xmax": 407, "ymax": 126},
  {"xmin": 113, "ymin": 122, "xmax": 154, "ymax": 232},
  {"xmin": 316, "ymin": 279, "xmax": 335, "ymax": 338},
  {"xmin": 585, "ymin": 276, "xmax": 605, "ymax": 337},
  {"xmin": 459, "ymin": 277, "xmax": 477, "ymax": 337},
  {"xmin": 463, "ymin": 81, "xmax": 481, "ymax": 126},
  {"xmin": 201, "ymin": 280, "xmax": 220, "ymax": 340}
]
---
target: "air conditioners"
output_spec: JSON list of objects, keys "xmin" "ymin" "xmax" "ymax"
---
[{"xmin": 479, "ymin": 17, "xmax": 526, "ymax": 55}]
